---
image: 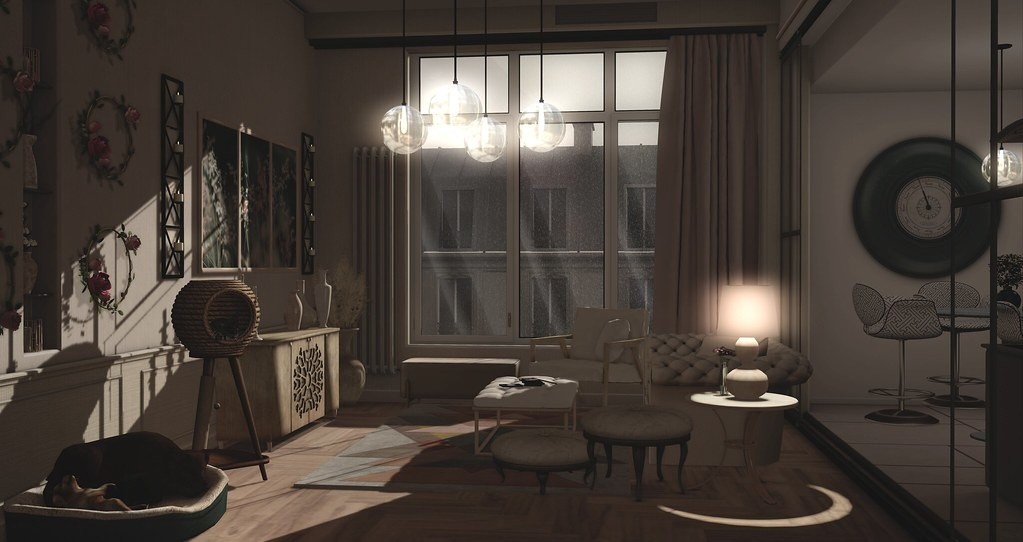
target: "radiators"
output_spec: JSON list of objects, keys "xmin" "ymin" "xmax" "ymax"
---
[{"xmin": 352, "ymin": 144, "xmax": 399, "ymax": 375}]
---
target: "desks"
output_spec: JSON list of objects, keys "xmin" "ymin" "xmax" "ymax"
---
[
  {"xmin": 212, "ymin": 326, "xmax": 342, "ymax": 451},
  {"xmin": 931, "ymin": 304, "xmax": 999, "ymax": 318},
  {"xmin": 690, "ymin": 391, "xmax": 798, "ymax": 507}
]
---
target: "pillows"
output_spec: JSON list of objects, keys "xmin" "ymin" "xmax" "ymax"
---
[{"xmin": 595, "ymin": 319, "xmax": 630, "ymax": 362}]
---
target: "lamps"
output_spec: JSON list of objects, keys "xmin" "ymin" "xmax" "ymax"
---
[
  {"xmin": 518, "ymin": 0, "xmax": 564, "ymax": 152},
  {"xmin": 429, "ymin": 0, "xmax": 482, "ymax": 140},
  {"xmin": 381, "ymin": 0, "xmax": 426, "ymax": 154},
  {"xmin": 465, "ymin": 0, "xmax": 505, "ymax": 163},
  {"xmin": 981, "ymin": 44, "xmax": 1021, "ymax": 186},
  {"xmin": 718, "ymin": 284, "xmax": 779, "ymax": 399}
]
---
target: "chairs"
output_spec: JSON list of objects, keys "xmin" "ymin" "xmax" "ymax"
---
[
  {"xmin": 527, "ymin": 305, "xmax": 650, "ymax": 406},
  {"xmin": 997, "ymin": 300, "xmax": 1023, "ymax": 347},
  {"xmin": 913, "ymin": 281, "xmax": 993, "ymax": 407},
  {"xmin": 851, "ymin": 284, "xmax": 943, "ymax": 423}
]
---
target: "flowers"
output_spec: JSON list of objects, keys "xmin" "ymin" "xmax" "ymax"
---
[
  {"xmin": 23, "ymin": 222, "xmax": 39, "ymax": 251},
  {"xmin": 0, "ymin": 53, "xmax": 63, "ymax": 168},
  {"xmin": 77, "ymin": 89, "xmax": 143, "ymax": 189},
  {"xmin": 79, "ymin": 224, "xmax": 140, "ymax": 315},
  {"xmin": 0, "ymin": 228, "xmax": 22, "ymax": 335},
  {"xmin": 80, "ymin": 0, "xmax": 136, "ymax": 62}
]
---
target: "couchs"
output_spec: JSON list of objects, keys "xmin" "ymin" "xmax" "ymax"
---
[{"xmin": 639, "ymin": 333, "xmax": 812, "ymax": 467}]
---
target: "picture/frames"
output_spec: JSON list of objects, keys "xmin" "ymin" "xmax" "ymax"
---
[
  {"xmin": 239, "ymin": 131, "xmax": 271, "ymax": 272},
  {"xmin": 197, "ymin": 110, "xmax": 239, "ymax": 273},
  {"xmin": 271, "ymin": 142, "xmax": 299, "ymax": 272}
]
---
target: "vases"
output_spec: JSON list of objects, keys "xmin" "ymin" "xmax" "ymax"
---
[
  {"xmin": 21, "ymin": 251, "xmax": 39, "ymax": 295},
  {"xmin": 23, "ymin": 134, "xmax": 39, "ymax": 189},
  {"xmin": 314, "ymin": 268, "xmax": 333, "ymax": 328},
  {"xmin": 339, "ymin": 327, "xmax": 366, "ymax": 406},
  {"xmin": 283, "ymin": 288, "xmax": 303, "ymax": 330}
]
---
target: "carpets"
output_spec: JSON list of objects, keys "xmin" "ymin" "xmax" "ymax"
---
[{"xmin": 292, "ymin": 401, "xmax": 632, "ymax": 498}]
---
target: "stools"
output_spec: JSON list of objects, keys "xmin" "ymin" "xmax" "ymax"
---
[
  {"xmin": 490, "ymin": 429, "xmax": 597, "ymax": 493},
  {"xmin": 579, "ymin": 405, "xmax": 693, "ymax": 501}
]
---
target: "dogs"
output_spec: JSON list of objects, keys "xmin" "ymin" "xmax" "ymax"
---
[{"xmin": 52, "ymin": 473, "xmax": 132, "ymax": 512}]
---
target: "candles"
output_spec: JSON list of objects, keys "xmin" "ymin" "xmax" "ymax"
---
[
  {"xmin": 309, "ymin": 247, "xmax": 315, "ymax": 256},
  {"xmin": 310, "ymin": 213, "xmax": 315, "ymax": 222},
  {"xmin": 175, "ymin": 141, "xmax": 184, "ymax": 153},
  {"xmin": 309, "ymin": 144, "xmax": 316, "ymax": 153},
  {"xmin": 174, "ymin": 91, "xmax": 183, "ymax": 103},
  {"xmin": 175, "ymin": 238, "xmax": 184, "ymax": 251},
  {"xmin": 174, "ymin": 190, "xmax": 184, "ymax": 202},
  {"xmin": 309, "ymin": 178, "xmax": 315, "ymax": 187}
]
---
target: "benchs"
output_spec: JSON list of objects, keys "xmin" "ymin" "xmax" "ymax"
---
[
  {"xmin": 401, "ymin": 354, "xmax": 521, "ymax": 408},
  {"xmin": 472, "ymin": 375, "xmax": 580, "ymax": 455}
]
